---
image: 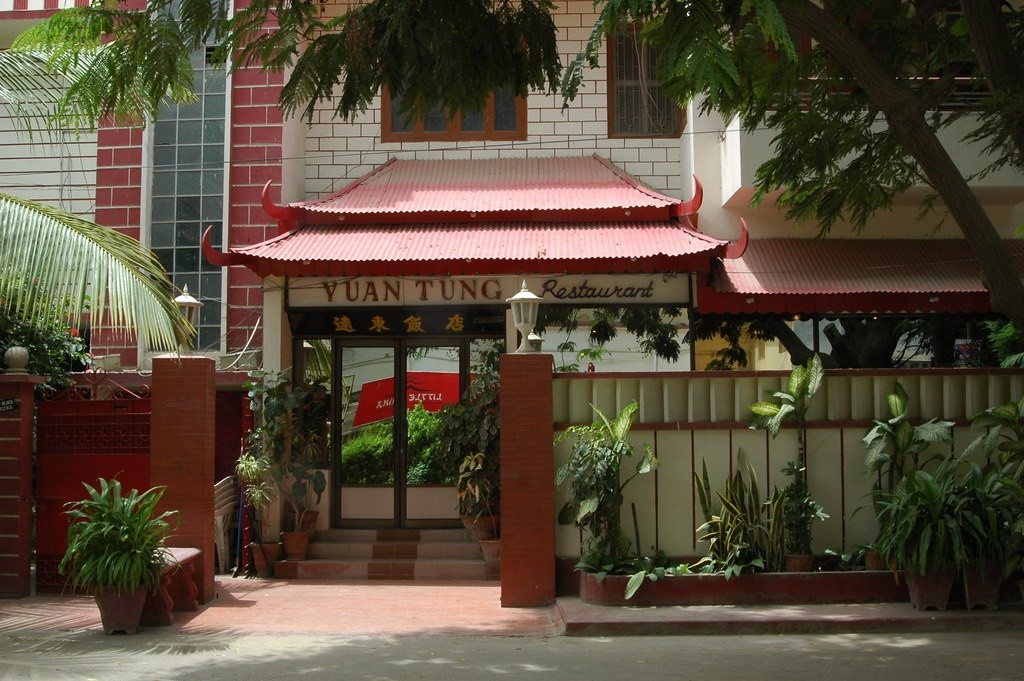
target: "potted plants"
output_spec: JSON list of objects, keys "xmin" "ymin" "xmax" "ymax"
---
[
  {"xmin": 54, "ymin": 467, "xmax": 182, "ymax": 635},
  {"xmin": 438, "ymin": 361, "xmax": 501, "ymax": 538},
  {"xmin": 848, "ymin": 453, "xmax": 988, "ymax": 612},
  {"xmin": 932, "ymin": 456, "xmax": 1024, "ymax": 614},
  {"xmin": 237, "ymin": 362, "xmax": 328, "ymax": 561},
  {"xmin": 454, "ymin": 452, "xmax": 504, "ymax": 580},
  {"xmin": 234, "ymin": 425, "xmax": 283, "ymax": 579},
  {"xmin": 779, "ymin": 457, "xmax": 831, "ymax": 572},
  {"xmin": 275, "ymin": 419, "xmax": 328, "ymax": 540}
]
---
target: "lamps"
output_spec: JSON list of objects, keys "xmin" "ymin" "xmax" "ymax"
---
[
  {"xmin": 170, "ymin": 283, "xmax": 204, "ymax": 355},
  {"xmin": 505, "ymin": 277, "xmax": 545, "ymax": 353}
]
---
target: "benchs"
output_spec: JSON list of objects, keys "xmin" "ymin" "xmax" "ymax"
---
[{"xmin": 142, "ymin": 543, "xmax": 202, "ymax": 628}]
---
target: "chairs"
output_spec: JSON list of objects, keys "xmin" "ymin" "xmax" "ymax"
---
[{"xmin": 213, "ymin": 473, "xmax": 239, "ymax": 575}]
